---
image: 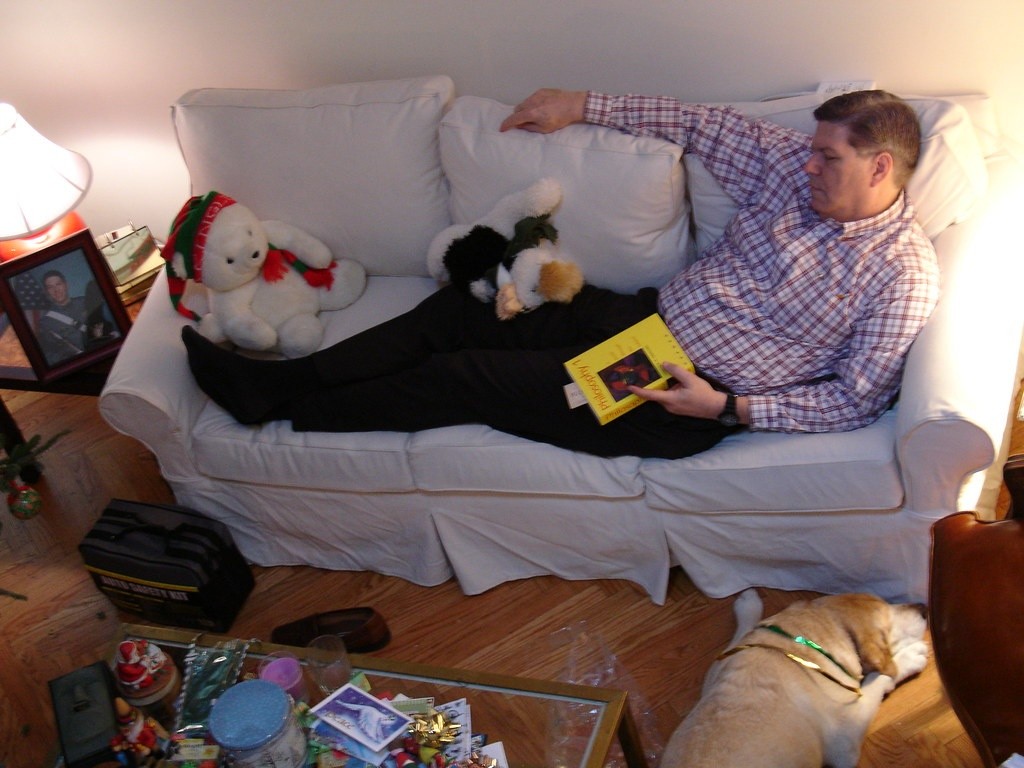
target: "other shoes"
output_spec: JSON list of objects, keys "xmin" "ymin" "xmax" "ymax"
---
[{"xmin": 272, "ymin": 607, "xmax": 393, "ymax": 655}]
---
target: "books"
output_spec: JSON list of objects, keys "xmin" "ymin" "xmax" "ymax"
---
[{"xmin": 564, "ymin": 313, "xmax": 696, "ymax": 425}]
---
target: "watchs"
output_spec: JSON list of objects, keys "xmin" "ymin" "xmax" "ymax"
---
[{"xmin": 716, "ymin": 392, "xmax": 741, "ymax": 427}]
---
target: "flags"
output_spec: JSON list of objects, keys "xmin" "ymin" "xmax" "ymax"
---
[{"xmin": 10, "ymin": 269, "xmax": 52, "ymax": 338}]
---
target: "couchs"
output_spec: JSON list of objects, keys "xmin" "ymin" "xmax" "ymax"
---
[{"xmin": 98, "ymin": 75, "xmax": 1024, "ymax": 606}]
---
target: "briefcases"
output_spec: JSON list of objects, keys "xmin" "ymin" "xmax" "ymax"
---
[{"xmin": 77, "ymin": 498, "xmax": 256, "ymax": 633}]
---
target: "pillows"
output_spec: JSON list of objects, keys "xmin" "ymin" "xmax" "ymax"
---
[
  {"xmin": 438, "ymin": 94, "xmax": 695, "ymax": 295},
  {"xmin": 171, "ymin": 75, "xmax": 455, "ymax": 277},
  {"xmin": 684, "ymin": 93, "xmax": 988, "ymax": 258}
]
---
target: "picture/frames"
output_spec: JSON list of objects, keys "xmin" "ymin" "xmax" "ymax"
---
[{"xmin": 0, "ymin": 229, "xmax": 132, "ymax": 384}]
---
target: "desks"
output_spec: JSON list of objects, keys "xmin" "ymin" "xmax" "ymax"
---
[
  {"xmin": 0, "ymin": 297, "xmax": 174, "ymax": 494},
  {"xmin": 41, "ymin": 622, "xmax": 649, "ymax": 768}
]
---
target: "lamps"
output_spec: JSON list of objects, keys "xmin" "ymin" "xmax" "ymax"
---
[{"xmin": 0, "ymin": 103, "xmax": 92, "ymax": 262}]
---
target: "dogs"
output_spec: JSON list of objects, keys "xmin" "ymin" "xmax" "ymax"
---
[{"xmin": 660, "ymin": 588, "xmax": 929, "ymax": 768}]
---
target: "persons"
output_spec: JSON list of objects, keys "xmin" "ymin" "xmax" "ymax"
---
[
  {"xmin": 35, "ymin": 270, "xmax": 120, "ymax": 370},
  {"xmin": 110, "ymin": 696, "xmax": 171, "ymax": 764},
  {"xmin": 116, "ymin": 639, "xmax": 155, "ymax": 690},
  {"xmin": 180, "ymin": 87, "xmax": 942, "ymax": 459}
]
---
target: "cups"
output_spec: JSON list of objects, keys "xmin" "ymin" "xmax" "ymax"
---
[
  {"xmin": 309, "ymin": 635, "xmax": 350, "ymax": 694},
  {"xmin": 258, "ymin": 651, "xmax": 310, "ymax": 706}
]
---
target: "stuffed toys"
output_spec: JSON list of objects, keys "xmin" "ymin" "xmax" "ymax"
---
[
  {"xmin": 160, "ymin": 190, "xmax": 367, "ymax": 360},
  {"xmin": 426, "ymin": 179, "xmax": 585, "ymax": 322}
]
